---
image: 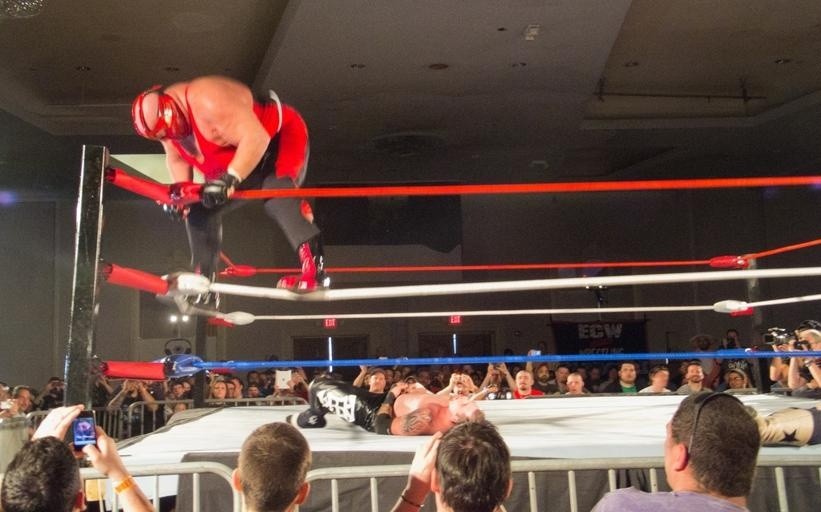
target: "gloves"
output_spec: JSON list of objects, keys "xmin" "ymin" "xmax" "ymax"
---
[
  {"xmin": 201, "ymin": 174, "xmax": 239, "ymax": 209},
  {"xmin": 162, "ymin": 203, "xmax": 189, "ymax": 222}
]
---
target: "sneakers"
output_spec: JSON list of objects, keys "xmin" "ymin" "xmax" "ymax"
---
[{"xmin": 286, "ymin": 408, "xmax": 327, "ymax": 429}]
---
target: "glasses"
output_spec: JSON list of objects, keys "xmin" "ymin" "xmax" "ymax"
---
[{"xmin": 687, "ymin": 391, "xmax": 741, "ymax": 455}]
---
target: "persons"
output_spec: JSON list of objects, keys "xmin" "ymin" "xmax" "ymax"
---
[
  {"xmin": 131, "ymin": 78, "xmax": 329, "ymax": 290},
  {"xmin": 589, "ymin": 390, "xmax": 760, "ymax": 511},
  {"xmin": 286, "ymin": 319, "xmax": 821, "ymax": 449},
  {"xmin": 1, "ymin": 355, "xmax": 286, "ymax": 436},
  {"xmin": 0, "ymin": 403, "xmax": 161, "ymax": 512},
  {"xmin": 393, "ymin": 415, "xmax": 515, "ymax": 512},
  {"xmin": 230, "ymin": 421, "xmax": 315, "ymax": 512}
]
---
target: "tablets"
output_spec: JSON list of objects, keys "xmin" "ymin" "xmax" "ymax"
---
[{"xmin": 274, "ymin": 368, "xmax": 292, "ymax": 391}]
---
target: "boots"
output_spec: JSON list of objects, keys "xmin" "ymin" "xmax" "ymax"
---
[
  {"xmin": 277, "ymin": 237, "xmax": 331, "ymax": 293},
  {"xmin": 154, "ymin": 273, "xmax": 222, "ymax": 317}
]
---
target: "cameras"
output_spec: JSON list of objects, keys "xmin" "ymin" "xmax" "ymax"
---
[
  {"xmin": 723, "ymin": 335, "xmax": 735, "ymax": 347},
  {"xmin": 530, "ymin": 350, "xmax": 541, "ymax": 358},
  {"xmin": 793, "ymin": 340, "xmax": 810, "ymax": 351}
]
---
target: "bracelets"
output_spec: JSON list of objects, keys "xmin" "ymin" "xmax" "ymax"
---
[
  {"xmin": 113, "ymin": 475, "xmax": 135, "ymax": 495},
  {"xmin": 399, "ymin": 493, "xmax": 425, "ymax": 509}
]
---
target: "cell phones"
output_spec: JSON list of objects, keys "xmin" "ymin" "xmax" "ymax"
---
[
  {"xmin": 51, "ymin": 380, "xmax": 62, "ymax": 388},
  {"xmin": 293, "ymin": 372, "xmax": 299, "ymax": 379},
  {"xmin": 70, "ymin": 410, "xmax": 95, "ymax": 450},
  {"xmin": 455, "ymin": 375, "xmax": 466, "ymax": 384}
]
